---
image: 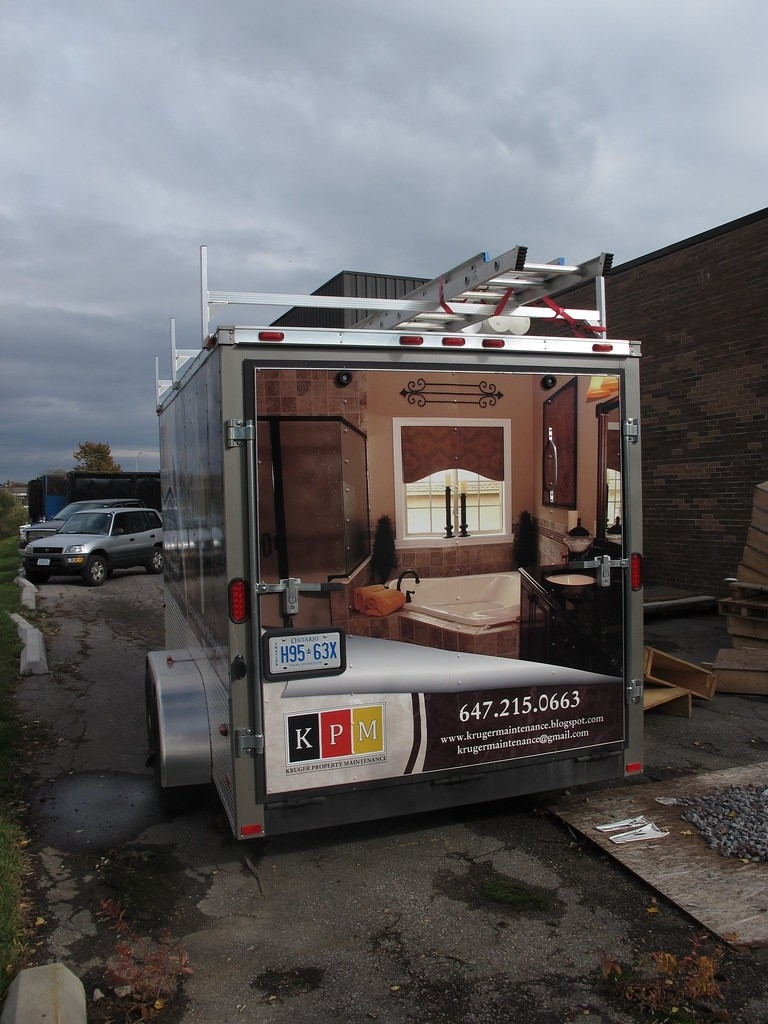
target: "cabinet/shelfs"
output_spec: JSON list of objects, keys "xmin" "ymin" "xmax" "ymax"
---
[{"xmin": 519, "ymin": 575, "xmax": 623, "ymax": 679}]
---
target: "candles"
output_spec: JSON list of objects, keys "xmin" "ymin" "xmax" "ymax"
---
[{"xmin": 445, "ymin": 471, "xmax": 451, "ymax": 485}]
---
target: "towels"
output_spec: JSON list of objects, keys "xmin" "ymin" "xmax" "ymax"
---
[{"xmin": 353, "ymin": 585, "xmax": 406, "ymax": 617}]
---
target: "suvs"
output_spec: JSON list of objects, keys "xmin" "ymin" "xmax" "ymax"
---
[
  {"xmin": 20, "ymin": 506, "xmax": 164, "ymax": 588},
  {"xmin": 161, "ymin": 505, "xmax": 227, "ymax": 582},
  {"xmin": 17, "ymin": 498, "xmax": 152, "ymax": 558}
]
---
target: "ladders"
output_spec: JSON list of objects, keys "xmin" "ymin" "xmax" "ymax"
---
[{"xmin": 346, "ymin": 244, "xmax": 614, "ymax": 333}]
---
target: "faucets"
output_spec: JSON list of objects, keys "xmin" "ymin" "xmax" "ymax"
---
[{"xmin": 396, "ymin": 569, "xmax": 420, "ymax": 592}]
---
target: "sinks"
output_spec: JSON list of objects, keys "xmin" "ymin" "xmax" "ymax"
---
[{"xmin": 545, "ymin": 573, "xmax": 596, "ymax": 586}]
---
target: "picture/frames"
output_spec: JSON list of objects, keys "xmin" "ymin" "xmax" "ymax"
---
[{"xmin": 540, "ymin": 376, "xmax": 577, "ymax": 510}]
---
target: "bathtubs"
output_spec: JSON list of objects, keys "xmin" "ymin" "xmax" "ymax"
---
[{"xmin": 385, "ymin": 570, "xmax": 521, "ymax": 626}]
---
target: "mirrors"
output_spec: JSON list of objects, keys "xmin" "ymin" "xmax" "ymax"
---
[
  {"xmin": 594, "ymin": 397, "xmax": 621, "ymax": 555},
  {"xmin": 460, "ymin": 480, "xmax": 468, "ymax": 494}
]
